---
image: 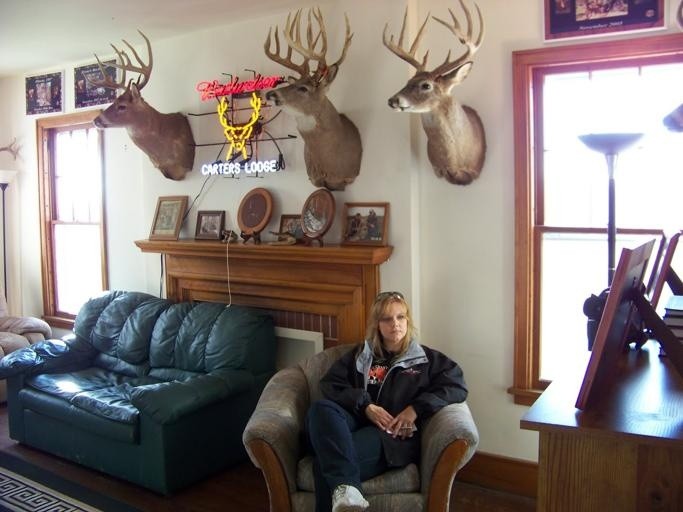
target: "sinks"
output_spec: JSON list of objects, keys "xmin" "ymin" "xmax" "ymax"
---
[{"xmin": 0, "ymin": 291, "xmax": 274, "ymax": 495}]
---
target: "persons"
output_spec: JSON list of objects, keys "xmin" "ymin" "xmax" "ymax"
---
[
  {"xmin": 365, "ymin": 208, "xmax": 381, "ymax": 242},
  {"xmin": 302, "ymin": 291, "xmax": 469, "ymax": 512},
  {"xmin": 343, "ymin": 212, "xmax": 364, "ymax": 241}
]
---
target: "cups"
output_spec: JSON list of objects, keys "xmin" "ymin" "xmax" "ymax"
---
[{"xmin": 377, "ymin": 292, "xmax": 404, "ymax": 303}]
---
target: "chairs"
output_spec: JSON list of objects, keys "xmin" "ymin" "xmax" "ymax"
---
[{"xmin": 332, "ymin": 485, "xmax": 369, "ymax": 512}]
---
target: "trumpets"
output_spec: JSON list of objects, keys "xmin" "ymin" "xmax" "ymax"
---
[
  {"xmin": 379, "ymin": 0, "xmax": 489, "ymax": 187},
  {"xmin": 262, "ymin": 2, "xmax": 365, "ymax": 195},
  {"xmin": 78, "ymin": 26, "xmax": 194, "ymax": 182}
]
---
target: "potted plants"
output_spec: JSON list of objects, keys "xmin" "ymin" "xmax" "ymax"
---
[
  {"xmin": 340, "ymin": 199, "xmax": 391, "ymax": 247},
  {"xmin": 149, "ymin": 195, "xmax": 188, "ymax": 241},
  {"xmin": 195, "ymin": 210, "xmax": 225, "ymax": 241},
  {"xmin": 575, "ymin": 239, "xmax": 683, "ymax": 410},
  {"xmin": 545, "ymin": 0, "xmax": 668, "ymax": 42},
  {"xmin": 644, "ymin": 231, "xmax": 683, "ymax": 329},
  {"xmin": 278, "ymin": 214, "xmax": 303, "ymax": 240}
]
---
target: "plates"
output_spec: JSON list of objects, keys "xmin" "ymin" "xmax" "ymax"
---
[{"xmin": 520, "ymin": 339, "xmax": 683, "ymax": 512}]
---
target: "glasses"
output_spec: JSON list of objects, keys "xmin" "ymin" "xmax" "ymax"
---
[
  {"xmin": 0, "ymin": 169, "xmax": 15, "ymax": 302},
  {"xmin": 577, "ymin": 133, "xmax": 638, "ymax": 285}
]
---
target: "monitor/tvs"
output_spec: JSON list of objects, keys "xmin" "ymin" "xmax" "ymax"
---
[{"xmin": 657, "ymin": 294, "xmax": 683, "ymax": 357}]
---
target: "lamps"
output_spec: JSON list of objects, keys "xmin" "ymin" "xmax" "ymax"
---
[
  {"xmin": 242, "ymin": 344, "xmax": 479, "ymax": 510},
  {"xmin": 0, "ymin": 291, "xmax": 52, "ymax": 405}
]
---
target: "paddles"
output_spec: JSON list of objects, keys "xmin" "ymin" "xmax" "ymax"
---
[{"xmin": 0, "ymin": 450, "xmax": 140, "ymax": 511}]
